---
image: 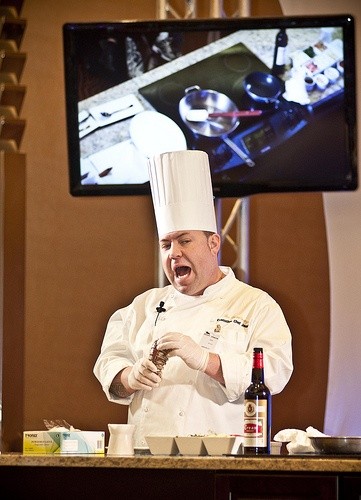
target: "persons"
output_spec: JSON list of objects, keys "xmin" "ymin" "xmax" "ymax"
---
[{"xmin": 93, "ymin": 150, "xmax": 294, "ymax": 450}]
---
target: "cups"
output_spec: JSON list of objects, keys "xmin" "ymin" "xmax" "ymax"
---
[{"xmin": 304, "ymin": 77, "xmax": 315, "ymax": 92}]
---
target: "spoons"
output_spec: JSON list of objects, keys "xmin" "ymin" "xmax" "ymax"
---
[
  {"xmin": 99, "ymin": 167, "xmax": 112, "ymax": 177},
  {"xmin": 80, "ymin": 173, "xmax": 88, "ymax": 180},
  {"xmin": 101, "ymin": 105, "xmax": 134, "ymax": 116}
]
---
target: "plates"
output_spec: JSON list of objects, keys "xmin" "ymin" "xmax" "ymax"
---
[
  {"xmin": 88, "ymin": 94, "xmax": 144, "ymax": 126},
  {"xmin": 79, "ymin": 110, "xmax": 99, "ymax": 139},
  {"xmin": 79, "ymin": 140, "xmax": 151, "ymax": 182},
  {"xmin": 129, "ymin": 111, "xmax": 186, "ymax": 157}
]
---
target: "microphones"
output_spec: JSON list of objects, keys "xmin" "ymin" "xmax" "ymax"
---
[{"xmin": 156, "ymin": 302, "xmax": 166, "ymax": 313}]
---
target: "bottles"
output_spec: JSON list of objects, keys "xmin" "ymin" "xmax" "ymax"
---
[
  {"xmin": 243, "ymin": 347, "xmax": 272, "ymax": 457},
  {"xmin": 272, "ymin": 29, "xmax": 288, "ymax": 73}
]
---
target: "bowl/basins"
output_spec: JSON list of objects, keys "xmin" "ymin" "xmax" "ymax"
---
[
  {"xmin": 269, "ymin": 441, "xmax": 282, "ymax": 455},
  {"xmin": 144, "ymin": 436, "xmax": 243, "ymax": 457},
  {"xmin": 242, "ymin": 72, "xmax": 284, "ymax": 103},
  {"xmin": 313, "ymin": 60, "xmax": 346, "ymax": 90},
  {"xmin": 308, "ymin": 436, "xmax": 361, "ymax": 454}
]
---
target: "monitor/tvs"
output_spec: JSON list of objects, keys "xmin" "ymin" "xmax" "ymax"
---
[{"xmin": 62, "ymin": 13, "xmax": 357, "ymax": 197}]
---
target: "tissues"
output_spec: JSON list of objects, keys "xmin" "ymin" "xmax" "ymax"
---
[{"xmin": 22, "ymin": 423, "xmax": 106, "ymax": 454}]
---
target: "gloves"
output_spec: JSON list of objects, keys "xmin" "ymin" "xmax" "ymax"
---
[
  {"xmin": 128, "ymin": 356, "xmax": 162, "ymax": 391},
  {"xmin": 157, "ymin": 331, "xmax": 209, "ymax": 373}
]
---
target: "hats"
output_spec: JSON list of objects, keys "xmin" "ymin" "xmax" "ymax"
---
[{"xmin": 147, "ymin": 150, "xmax": 217, "ymax": 237}]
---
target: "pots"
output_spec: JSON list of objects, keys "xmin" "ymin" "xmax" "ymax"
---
[{"xmin": 179, "ymin": 85, "xmax": 255, "ymax": 168}]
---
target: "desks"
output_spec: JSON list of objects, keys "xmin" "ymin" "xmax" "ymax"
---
[{"xmin": 0, "ymin": 453, "xmax": 361, "ymax": 500}]
